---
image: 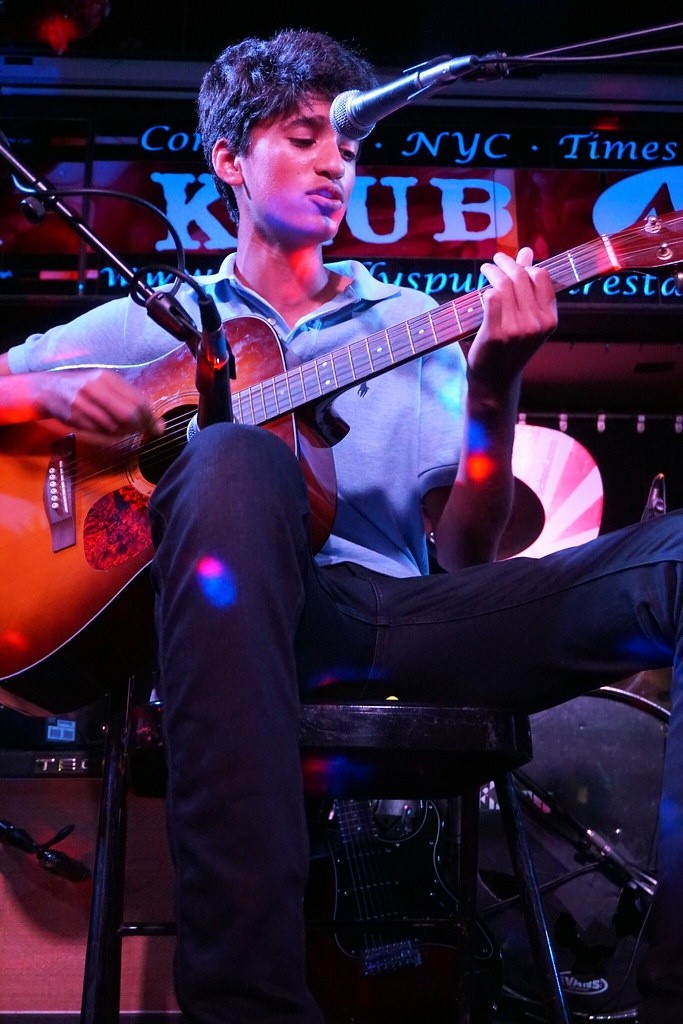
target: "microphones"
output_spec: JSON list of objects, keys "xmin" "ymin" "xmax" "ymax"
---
[
  {"xmin": 329, "ymin": 56, "xmax": 480, "ymax": 140},
  {"xmin": 185, "ymin": 295, "xmax": 240, "ymax": 443},
  {"xmin": 38, "ymin": 847, "xmax": 92, "ymax": 883}
]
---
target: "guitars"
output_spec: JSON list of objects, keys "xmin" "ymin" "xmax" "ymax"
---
[
  {"xmin": 306, "ymin": 796, "xmax": 504, "ymax": 1024},
  {"xmin": 0, "ymin": 208, "xmax": 683, "ymax": 716}
]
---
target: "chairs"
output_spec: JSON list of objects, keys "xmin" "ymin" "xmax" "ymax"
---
[{"xmin": 79, "ymin": 670, "xmax": 571, "ymax": 1024}]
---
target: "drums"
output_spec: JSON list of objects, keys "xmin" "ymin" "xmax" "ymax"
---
[{"xmin": 446, "ymin": 686, "xmax": 671, "ymax": 1023}]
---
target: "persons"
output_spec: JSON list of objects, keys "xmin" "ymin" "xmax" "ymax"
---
[{"xmin": 0, "ymin": 27, "xmax": 683, "ymax": 1024}]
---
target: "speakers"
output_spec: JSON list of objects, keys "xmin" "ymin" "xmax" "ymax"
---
[{"xmin": 0, "ymin": 749, "xmax": 188, "ymax": 1024}]
